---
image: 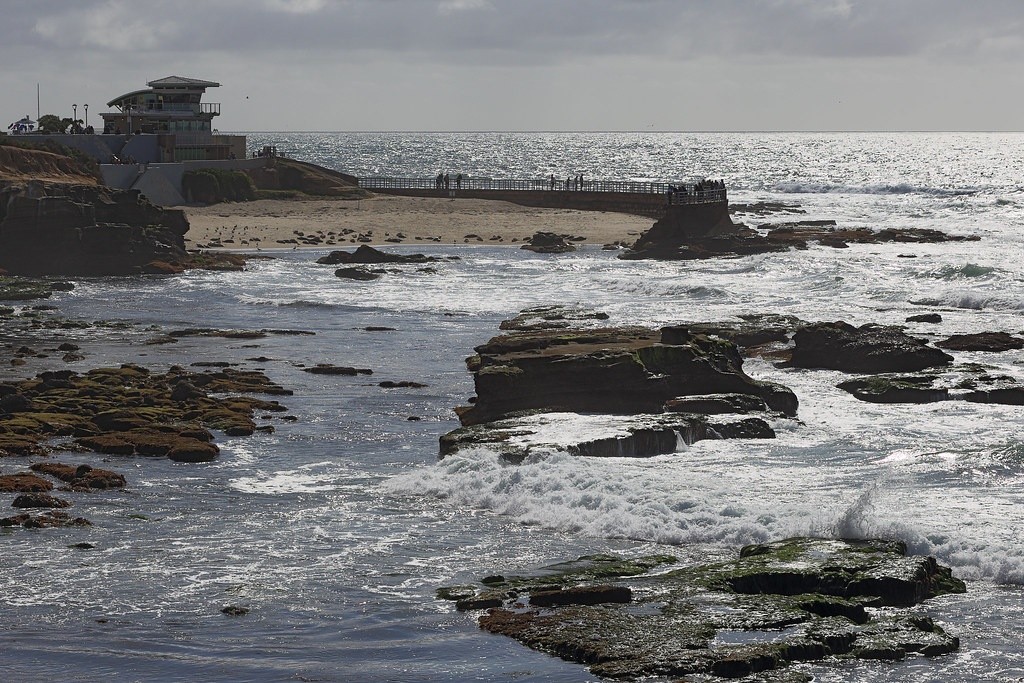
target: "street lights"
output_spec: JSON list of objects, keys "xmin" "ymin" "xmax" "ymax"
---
[
  {"xmin": 127, "ymin": 103, "xmax": 131, "ymax": 134},
  {"xmin": 71, "ymin": 104, "xmax": 78, "ymax": 120},
  {"xmin": 83, "ymin": 103, "xmax": 89, "ymax": 128}
]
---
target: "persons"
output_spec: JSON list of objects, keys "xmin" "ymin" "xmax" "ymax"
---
[
  {"xmin": 566, "ymin": 177, "xmax": 572, "ymax": 190},
  {"xmin": 574, "ymin": 176, "xmax": 579, "ymax": 192},
  {"xmin": 551, "ymin": 174, "xmax": 556, "ymax": 190},
  {"xmin": 436, "ymin": 174, "xmax": 444, "ymax": 189},
  {"xmin": 456, "ymin": 174, "xmax": 462, "ymax": 188},
  {"xmin": 444, "ymin": 174, "xmax": 449, "ymax": 189},
  {"xmin": 667, "ymin": 184, "xmax": 673, "ymax": 204},
  {"xmin": 580, "ymin": 174, "xmax": 584, "ymax": 191},
  {"xmin": 670, "ymin": 179, "xmax": 725, "ymax": 202}
]
